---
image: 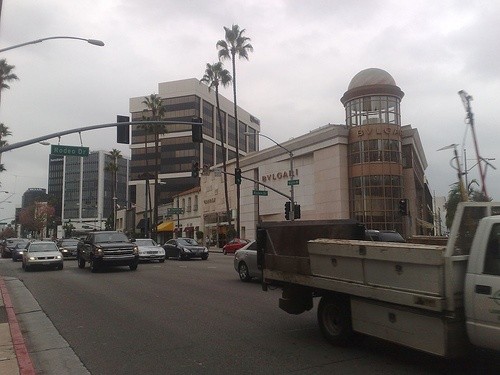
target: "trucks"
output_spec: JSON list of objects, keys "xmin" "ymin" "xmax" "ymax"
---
[{"xmin": 256, "ymin": 201, "xmax": 499, "ymax": 360}]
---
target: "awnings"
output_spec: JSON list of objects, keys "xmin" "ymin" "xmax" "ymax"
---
[
  {"xmin": 136, "ymin": 218, "xmax": 150, "ymax": 228},
  {"xmin": 152, "ymin": 220, "xmax": 174, "ymax": 232}
]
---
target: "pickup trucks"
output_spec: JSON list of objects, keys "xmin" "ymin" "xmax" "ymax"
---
[{"xmin": 76, "ymin": 231, "xmax": 138, "ymax": 273}]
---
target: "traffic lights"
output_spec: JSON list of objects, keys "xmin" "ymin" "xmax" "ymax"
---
[
  {"xmin": 192, "ymin": 160, "xmax": 199, "ymax": 178},
  {"xmin": 285, "ymin": 202, "xmax": 290, "ymax": 220},
  {"xmin": 400, "ymin": 199, "xmax": 407, "ymax": 216},
  {"xmin": 235, "ymin": 168, "xmax": 241, "ymax": 184}
]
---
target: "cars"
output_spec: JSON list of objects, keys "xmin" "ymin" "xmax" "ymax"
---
[
  {"xmin": 22, "ymin": 241, "xmax": 64, "ymax": 271},
  {"xmin": 364, "ymin": 229, "xmax": 404, "ymax": 243},
  {"xmin": 234, "ymin": 240, "xmax": 261, "ymax": 281},
  {"xmin": 10, "ymin": 242, "xmax": 26, "ymax": 261},
  {"xmin": 222, "ymin": 238, "xmax": 250, "ymax": 255},
  {"xmin": 132, "ymin": 239, "xmax": 166, "ymax": 263},
  {"xmin": 57, "ymin": 238, "xmax": 80, "ymax": 257},
  {"xmin": 2, "ymin": 238, "xmax": 20, "ymax": 258},
  {"xmin": 162, "ymin": 238, "xmax": 208, "ymax": 261}
]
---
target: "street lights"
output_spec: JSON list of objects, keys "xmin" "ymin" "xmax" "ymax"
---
[{"xmin": 244, "ymin": 132, "xmax": 294, "ymax": 221}]
